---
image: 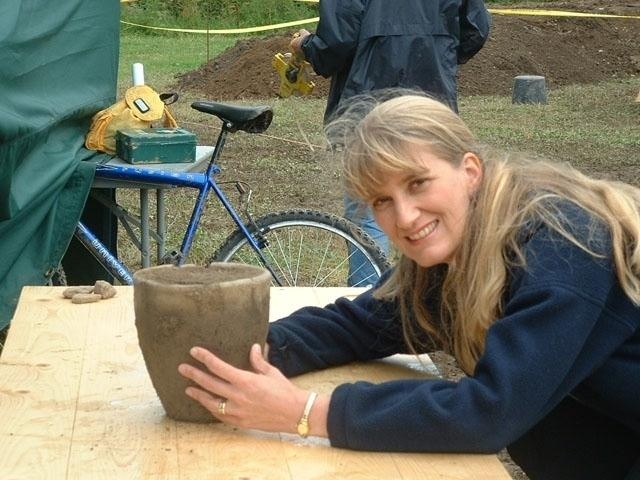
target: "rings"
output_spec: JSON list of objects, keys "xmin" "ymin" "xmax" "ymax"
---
[{"xmin": 219, "ymin": 400, "xmax": 226, "ymax": 416}]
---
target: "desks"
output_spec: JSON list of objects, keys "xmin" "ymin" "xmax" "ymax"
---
[
  {"xmin": 0, "ymin": 284, "xmax": 513, "ymax": 480},
  {"xmin": 90, "ymin": 145, "xmax": 218, "ymax": 269}
]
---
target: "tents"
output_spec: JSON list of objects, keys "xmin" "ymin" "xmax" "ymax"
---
[{"xmin": 1, "ymin": 0, "xmax": 122, "ymax": 330}]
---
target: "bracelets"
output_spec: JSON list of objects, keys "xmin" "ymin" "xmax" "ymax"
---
[{"xmin": 299, "ymin": 391, "xmax": 318, "ymax": 439}]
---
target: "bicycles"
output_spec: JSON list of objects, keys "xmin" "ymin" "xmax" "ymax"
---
[{"xmin": 44, "ymin": 101, "xmax": 389, "ymax": 288}]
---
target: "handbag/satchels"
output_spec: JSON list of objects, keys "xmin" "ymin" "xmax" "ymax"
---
[{"xmin": 85, "ymin": 85, "xmax": 178, "ymax": 156}]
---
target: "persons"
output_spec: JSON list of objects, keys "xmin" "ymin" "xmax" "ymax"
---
[
  {"xmin": 291, "ymin": 1, "xmax": 492, "ymax": 287},
  {"xmin": 177, "ymin": 84, "xmax": 640, "ymax": 479}
]
